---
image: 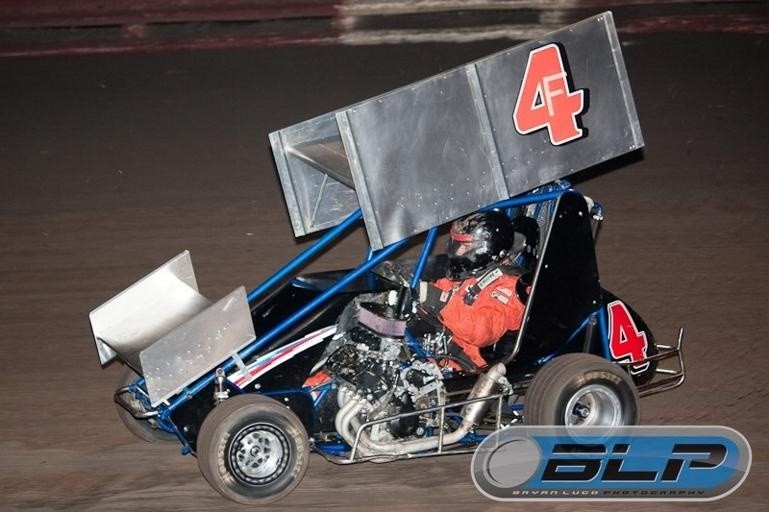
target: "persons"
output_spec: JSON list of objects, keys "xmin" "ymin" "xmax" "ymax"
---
[{"xmin": 301, "ymin": 210, "xmax": 536, "ymax": 456}]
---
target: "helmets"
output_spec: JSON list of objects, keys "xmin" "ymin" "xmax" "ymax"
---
[{"xmin": 445, "ymin": 210, "xmax": 514, "ymax": 279}]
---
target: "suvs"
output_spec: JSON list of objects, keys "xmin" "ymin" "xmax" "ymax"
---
[{"xmin": 89, "ymin": 9, "xmax": 687, "ymax": 507}]
